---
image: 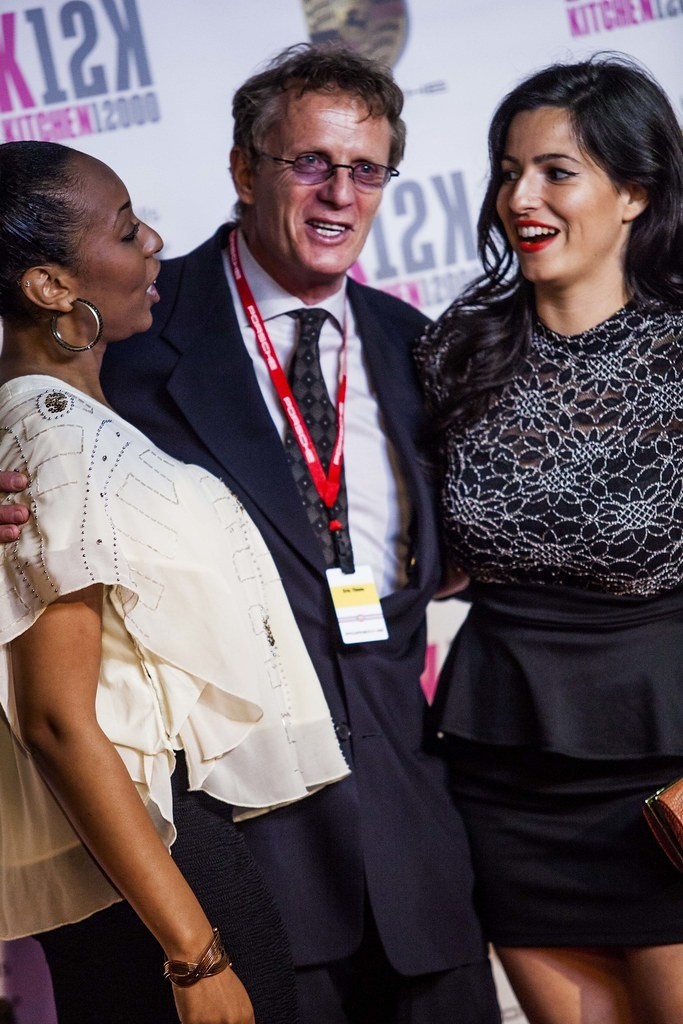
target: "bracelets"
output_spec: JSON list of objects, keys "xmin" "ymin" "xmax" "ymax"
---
[{"xmin": 161, "ymin": 927, "xmax": 230, "ymax": 987}]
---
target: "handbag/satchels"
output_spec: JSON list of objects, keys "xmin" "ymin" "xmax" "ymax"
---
[{"xmin": 642, "ymin": 776, "xmax": 683, "ymax": 873}]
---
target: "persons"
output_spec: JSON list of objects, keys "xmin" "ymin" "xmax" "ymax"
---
[
  {"xmin": 416, "ymin": 53, "xmax": 683, "ymax": 1024},
  {"xmin": 0, "ymin": 50, "xmax": 509, "ymax": 1024},
  {"xmin": 0, "ymin": 140, "xmax": 399, "ymax": 1023}
]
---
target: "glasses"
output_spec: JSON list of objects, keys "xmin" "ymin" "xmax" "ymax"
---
[{"xmin": 260, "ymin": 151, "xmax": 400, "ymax": 193}]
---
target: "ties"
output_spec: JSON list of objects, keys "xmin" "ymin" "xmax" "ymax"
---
[{"xmin": 285, "ymin": 309, "xmax": 356, "ymax": 574}]
